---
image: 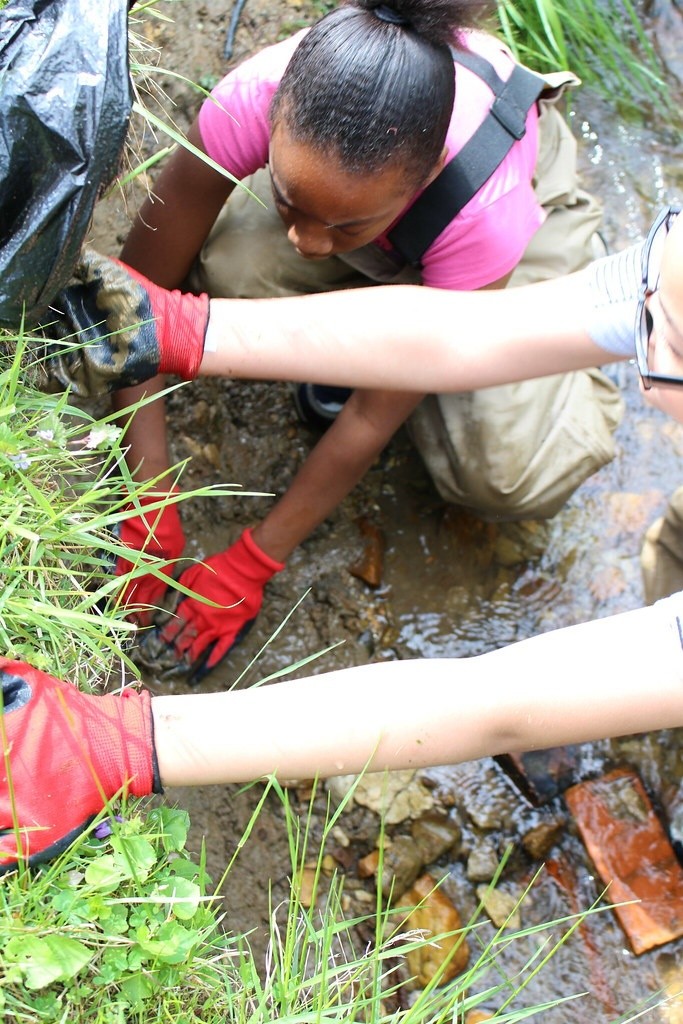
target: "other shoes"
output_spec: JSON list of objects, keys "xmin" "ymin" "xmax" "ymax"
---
[{"xmin": 292, "ymin": 378, "xmax": 353, "ymax": 436}]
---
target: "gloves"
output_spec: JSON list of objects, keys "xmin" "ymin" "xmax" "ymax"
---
[
  {"xmin": 34, "ymin": 238, "xmax": 210, "ymax": 398},
  {"xmin": 98, "ymin": 484, "xmax": 186, "ymax": 633},
  {"xmin": 129, "ymin": 526, "xmax": 286, "ymax": 688},
  {"xmin": 0, "ymin": 655, "xmax": 164, "ymax": 877}
]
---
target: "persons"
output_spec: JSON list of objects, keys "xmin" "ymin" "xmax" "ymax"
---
[
  {"xmin": 0, "ymin": 205, "xmax": 683, "ymax": 874},
  {"xmin": 113, "ymin": 0, "xmax": 625, "ymax": 668}
]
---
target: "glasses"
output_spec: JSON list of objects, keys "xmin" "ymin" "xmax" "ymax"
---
[{"xmin": 635, "ymin": 207, "xmax": 683, "ymax": 390}]
